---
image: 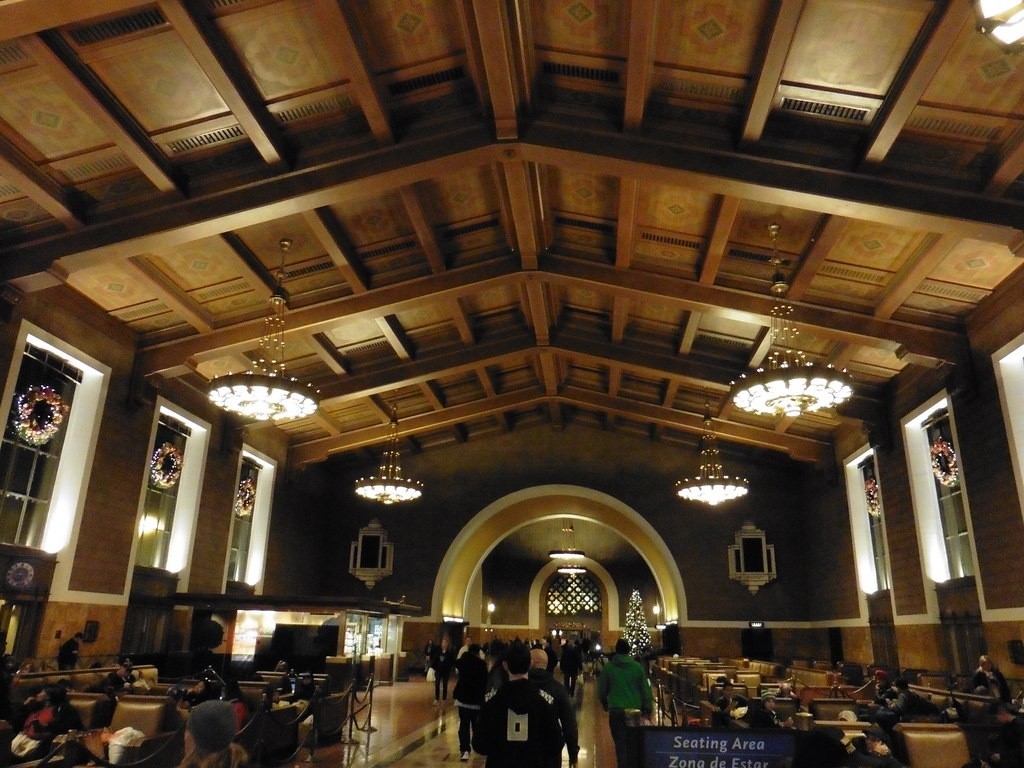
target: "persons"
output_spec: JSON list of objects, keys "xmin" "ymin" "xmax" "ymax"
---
[
  {"xmin": 0, "ymin": 631, "xmax": 317, "ymax": 768},
  {"xmin": 710, "ymin": 655, "xmax": 1024, "ymax": 768},
  {"xmin": 424, "ymin": 634, "xmax": 652, "ymax": 768}
]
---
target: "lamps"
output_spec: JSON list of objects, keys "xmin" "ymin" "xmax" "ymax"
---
[
  {"xmin": 354, "ymin": 388, "xmax": 424, "ymax": 504},
  {"xmin": 206, "ymin": 239, "xmax": 320, "ymax": 419},
  {"xmin": 675, "ymin": 385, "xmax": 750, "ymax": 505},
  {"xmin": 549, "ymin": 518, "xmax": 586, "ymax": 574},
  {"xmin": 729, "ymin": 223, "xmax": 855, "ymax": 416},
  {"xmin": 971, "ymin": 0, "xmax": 1024, "ymax": 56}
]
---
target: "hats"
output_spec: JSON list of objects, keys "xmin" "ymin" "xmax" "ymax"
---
[
  {"xmin": 187, "ymin": 700, "xmax": 235, "ymax": 749},
  {"xmin": 838, "ymin": 709, "xmax": 857, "ymax": 721},
  {"xmin": 863, "ymin": 727, "xmax": 892, "ymax": 750},
  {"xmin": 875, "ymin": 670, "xmax": 888, "ymax": 678},
  {"xmin": 616, "ymin": 639, "xmax": 631, "ymax": 654},
  {"xmin": 761, "ymin": 689, "xmax": 775, "ymax": 701}
]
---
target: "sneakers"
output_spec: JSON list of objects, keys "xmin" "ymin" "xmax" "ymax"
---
[{"xmin": 460, "ymin": 751, "xmax": 470, "ymax": 760}]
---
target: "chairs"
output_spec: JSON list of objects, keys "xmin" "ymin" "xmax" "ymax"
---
[
  {"xmin": 649, "ymin": 655, "xmax": 1024, "ymax": 768},
  {"xmin": 0, "ymin": 661, "xmax": 348, "ymax": 768}
]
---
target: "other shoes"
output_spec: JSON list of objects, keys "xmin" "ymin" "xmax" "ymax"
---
[
  {"xmin": 442, "ymin": 700, "xmax": 447, "ymax": 706},
  {"xmin": 433, "ymin": 700, "xmax": 439, "ymax": 706}
]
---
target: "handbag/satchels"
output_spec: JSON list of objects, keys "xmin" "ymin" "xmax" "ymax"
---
[
  {"xmin": 81, "ymin": 727, "xmax": 114, "ymax": 766},
  {"xmin": 426, "ymin": 668, "xmax": 435, "ymax": 682},
  {"xmin": 578, "ymin": 671, "xmax": 584, "ymax": 686},
  {"xmin": 108, "ymin": 736, "xmax": 146, "ymax": 764},
  {"xmin": 12, "ymin": 726, "xmax": 42, "ymax": 759}
]
